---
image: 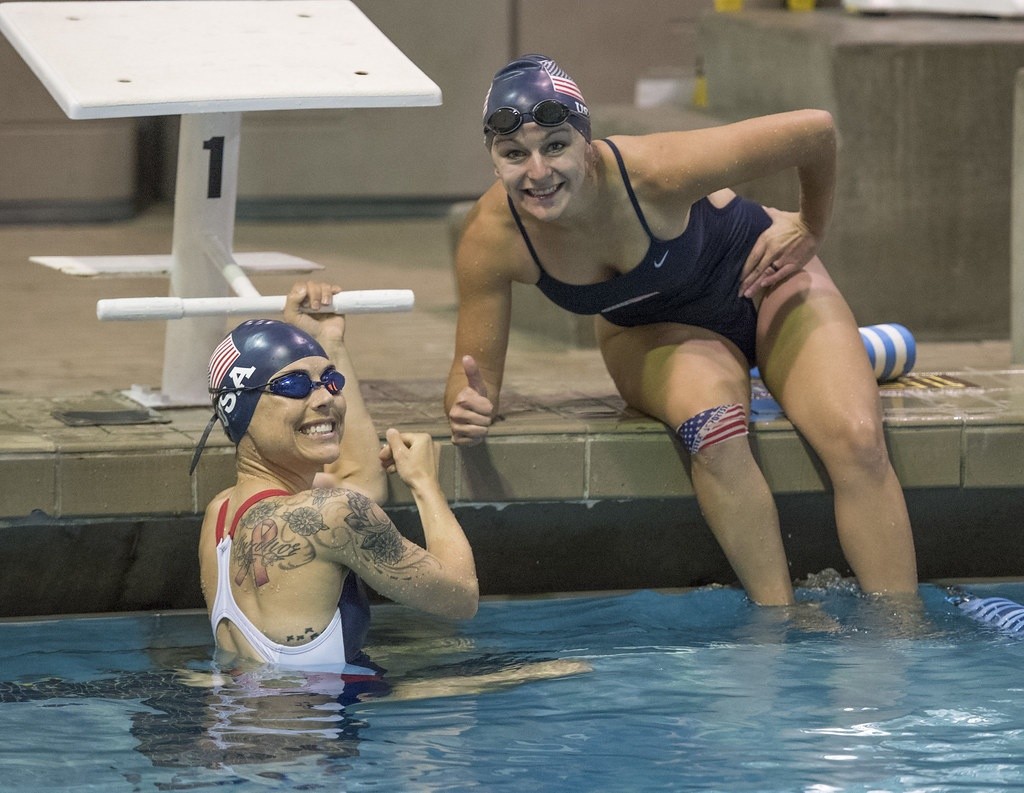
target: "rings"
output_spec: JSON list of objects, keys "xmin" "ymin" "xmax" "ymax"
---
[{"xmin": 770, "ymin": 263, "xmax": 779, "ymax": 272}]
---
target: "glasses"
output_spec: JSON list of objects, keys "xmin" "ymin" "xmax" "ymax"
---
[
  {"xmin": 485, "ymin": 98, "xmax": 570, "ymax": 136},
  {"xmin": 270, "ymin": 368, "xmax": 344, "ymax": 399}
]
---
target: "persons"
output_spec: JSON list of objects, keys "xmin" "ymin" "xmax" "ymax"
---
[
  {"xmin": 188, "ymin": 281, "xmax": 479, "ymax": 664},
  {"xmin": 444, "ymin": 53, "xmax": 919, "ymax": 605}
]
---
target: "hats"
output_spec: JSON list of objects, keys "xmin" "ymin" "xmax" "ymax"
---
[
  {"xmin": 483, "ymin": 54, "xmax": 591, "ymax": 153},
  {"xmin": 207, "ymin": 318, "xmax": 329, "ymax": 448}
]
